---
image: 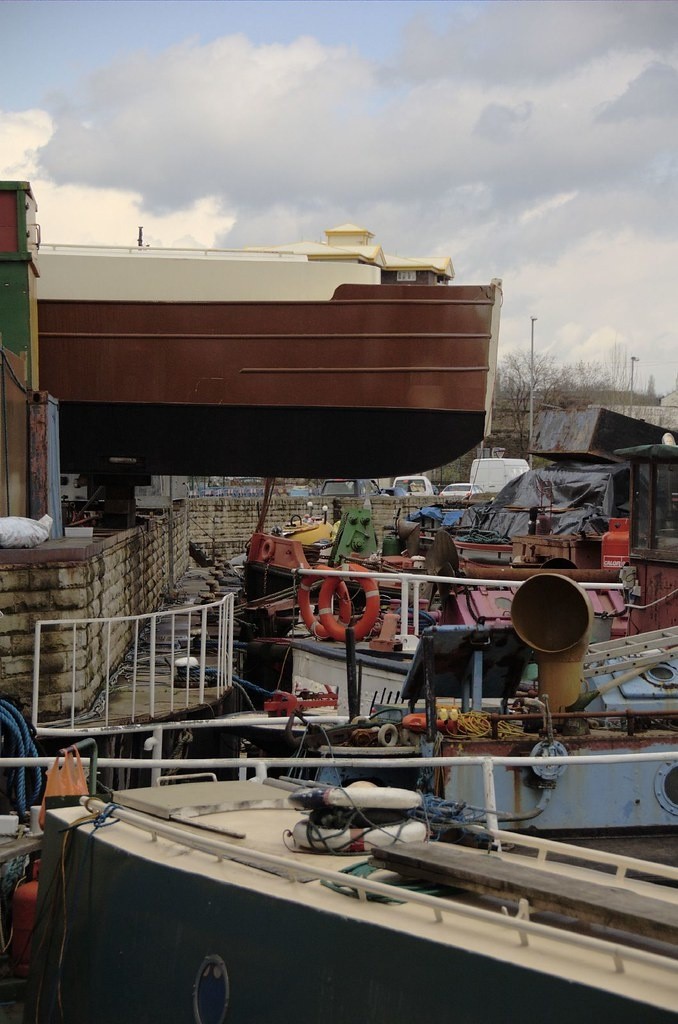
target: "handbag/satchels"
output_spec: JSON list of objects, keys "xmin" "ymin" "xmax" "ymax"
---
[{"xmin": 39, "ymin": 745, "xmax": 89, "ymax": 830}]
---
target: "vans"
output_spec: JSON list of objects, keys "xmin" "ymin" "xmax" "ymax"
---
[
  {"xmin": 319, "ymin": 478, "xmax": 379, "ymax": 498},
  {"xmin": 470, "ymin": 458, "xmax": 531, "ymax": 496},
  {"xmin": 392, "ymin": 476, "xmax": 435, "ymax": 495}
]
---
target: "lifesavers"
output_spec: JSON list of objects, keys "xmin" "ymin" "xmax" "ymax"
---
[
  {"xmin": 298, "ymin": 565, "xmax": 351, "ymax": 638},
  {"xmin": 319, "ymin": 563, "xmax": 380, "ymax": 640},
  {"xmin": 288, "ymin": 787, "xmax": 423, "ymax": 811},
  {"xmin": 292, "ymin": 818, "xmax": 427, "ymax": 849}
]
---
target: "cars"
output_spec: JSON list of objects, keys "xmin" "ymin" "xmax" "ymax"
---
[{"xmin": 439, "ymin": 482, "xmax": 484, "ymax": 497}]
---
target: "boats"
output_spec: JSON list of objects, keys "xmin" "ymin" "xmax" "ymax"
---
[{"xmin": 0, "ymin": 468, "xmax": 678, "ymax": 1024}]
